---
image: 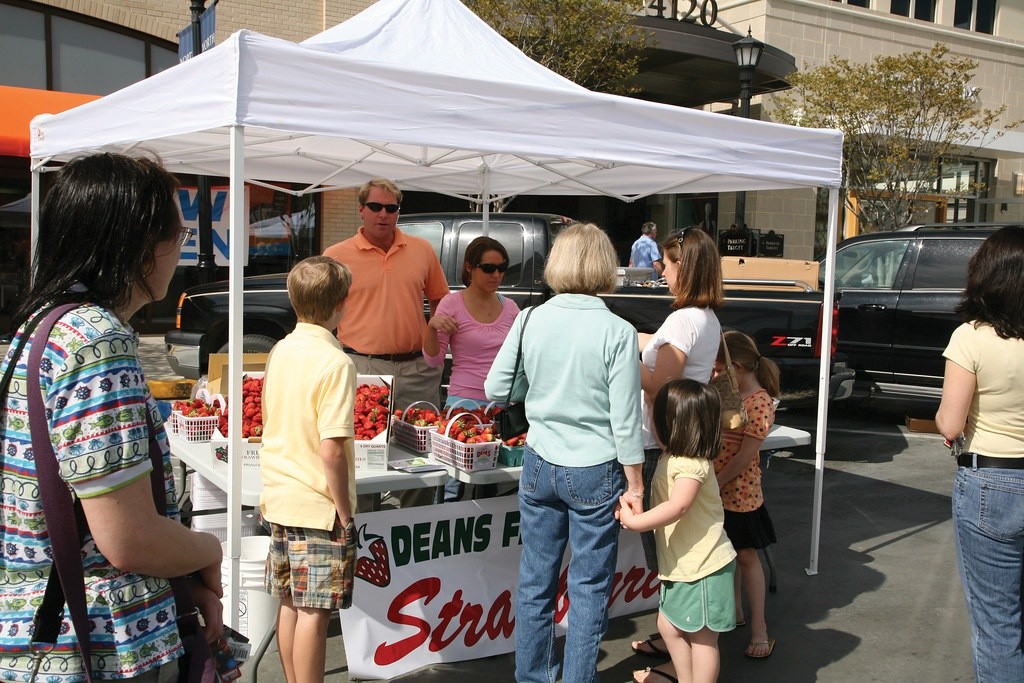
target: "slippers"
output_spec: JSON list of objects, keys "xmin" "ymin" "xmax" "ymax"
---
[
  {"xmin": 744, "ymin": 639, "xmax": 775, "ymax": 658},
  {"xmin": 736, "ymin": 620, "xmax": 745, "ymax": 626},
  {"xmin": 632, "ymin": 660, "xmax": 679, "ymax": 683},
  {"xmin": 631, "ymin": 638, "xmax": 669, "ymax": 657}
]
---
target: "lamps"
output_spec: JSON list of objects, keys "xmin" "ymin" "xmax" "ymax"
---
[
  {"xmin": 1001, "ymin": 203, "xmax": 1007, "ymax": 213},
  {"xmin": 1012, "ymin": 171, "xmax": 1024, "ymax": 196}
]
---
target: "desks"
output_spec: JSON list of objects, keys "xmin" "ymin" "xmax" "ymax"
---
[
  {"xmin": 391, "ymin": 422, "xmax": 811, "ymax": 593},
  {"xmin": 157, "ymin": 399, "xmax": 451, "ymax": 683}
]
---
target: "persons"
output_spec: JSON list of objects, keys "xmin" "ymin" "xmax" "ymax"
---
[
  {"xmin": 423, "ymin": 236, "xmax": 528, "ymax": 501},
  {"xmin": 322, "ymin": 179, "xmax": 451, "ymax": 514},
  {"xmin": 0, "ymin": 148, "xmax": 223, "ymax": 683},
  {"xmin": 711, "ymin": 330, "xmax": 778, "ymax": 660},
  {"xmin": 484, "ymin": 222, "xmax": 645, "ymax": 683},
  {"xmin": 618, "ymin": 377, "xmax": 738, "ymax": 683},
  {"xmin": 629, "ymin": 222, "xmax": 663, "ymax": 276},
  {"xmin": 935, "ymin": 225, "xmax": 1024, "ymax": 683},
  {"xmin": 632, "ymin": 225, "xmax": 723, "ymax": 683},
  {"xmin": 259, "ymin": 255, "xmax": 358, "ymax": 683}
]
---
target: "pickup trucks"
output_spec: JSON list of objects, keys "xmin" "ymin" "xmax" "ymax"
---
[{"xmin": 172, "ymin": 212, "xmax": 853, "ymax": 418}]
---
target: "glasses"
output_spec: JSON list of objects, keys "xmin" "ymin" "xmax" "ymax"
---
[
  {"xmin": 475, "ymin": 261, "xmax": 509, "ymax": 274},
  {"xmin": 177, "ymin": 227, "xmax": 193, "ymax": 246},
  {"xmin": 675, "ymin": 225, "xmax": 693, "ymax": 253},
  {"xmin": 364, "ymin": 202, "xmax": 401, "ymax": 213}
]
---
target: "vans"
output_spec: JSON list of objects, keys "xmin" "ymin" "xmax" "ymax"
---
[{"xmin": 781, "ymin": 224, "xmax": 1024, "ymax": 411}]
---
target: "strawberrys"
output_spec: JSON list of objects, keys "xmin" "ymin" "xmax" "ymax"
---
[
  {"xmin": 167, "ymin": 397, "xmax": 222, "ymax": 436},
  {"xmin": 353, "ymin": 383, "xmax": 389, "ymax": 442},
  {"xmin": 395, "ymin": 405, "xmax": 527, "ymax": 469},
  {"xmin": 216, "ymin": 374, "xmax": 262, "ymax": 438}
]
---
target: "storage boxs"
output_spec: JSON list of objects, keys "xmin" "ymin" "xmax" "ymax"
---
[{"xmin": 204, "ymin": 353, "xmax": 393, "ymax": 476}]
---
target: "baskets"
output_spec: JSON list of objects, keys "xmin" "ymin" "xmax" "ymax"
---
[
  {"xmin": 167, "ymin": 404, "xmax": 219, "ymax": 443},
  {"xmin": 392, "ymin": 399, "xmax": 528, "ymax": 473}
]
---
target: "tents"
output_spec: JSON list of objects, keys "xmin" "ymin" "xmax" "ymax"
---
[{"xmin": 29, "ymin": 0, "xmax": 845, "ymax": 576}]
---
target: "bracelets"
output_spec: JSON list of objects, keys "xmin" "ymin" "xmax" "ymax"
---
[{"xmin": 628, "ymin": 489, "xmax": 644, "ymax": 497}]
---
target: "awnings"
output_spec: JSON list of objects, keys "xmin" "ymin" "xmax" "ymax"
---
[{"xmin": 0, "ymin": 86, "xmax": 107, "ymax": 158}]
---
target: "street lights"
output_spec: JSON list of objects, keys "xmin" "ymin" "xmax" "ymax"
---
[{"xmin": 722, "ymin": 24, "xmax": 765, "ymax": 260}]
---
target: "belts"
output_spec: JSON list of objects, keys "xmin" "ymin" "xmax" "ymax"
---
[
  {"xmin": 957, "ymin": 453, "xmax": 1024, "ymax": 469},
  {"xmin": 342, "ymin": 347, "xmax": 424, "ymax": 363}
]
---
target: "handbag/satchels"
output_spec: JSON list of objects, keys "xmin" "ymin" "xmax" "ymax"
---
[
  {"xmin": 709, "ymin": 327, "xmax": 749, "ymax": 430},
  {"xmin": 177, "ymin": 651, "xmax": 218, "ymax": 683},
  {"xmin": 493, "ymin": 401, "xmax": 529, "ymax": 442}
]
